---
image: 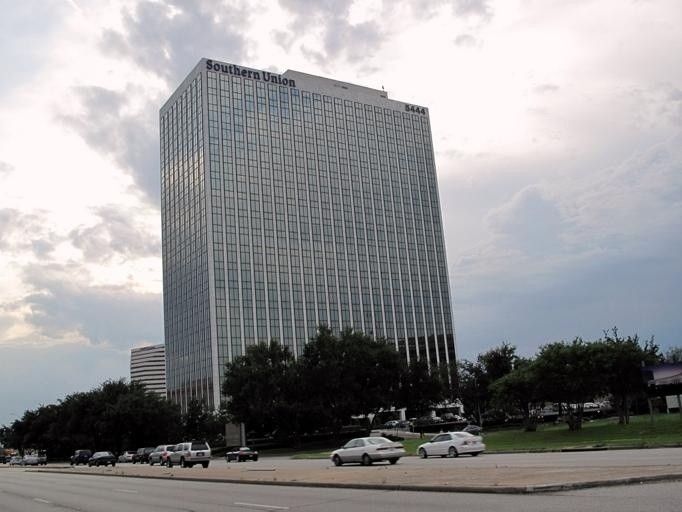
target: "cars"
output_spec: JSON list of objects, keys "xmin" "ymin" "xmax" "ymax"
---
[
  {"xmin": 0, "ymin": 455, "xmax": 47, "ymax": 465},
  {"xmin": 535, "ymin": 400, "xmax": 613, "ymax": 413},
  {"xmin": 70, "ymin": 441, "xmax": 211, "ymax": 468},
  {"xmin": 330, "ymin": 436, "xmax": 405, "ymax": 466},
  {"xmin": 225, "ymin": 446, "xmax": 259, "ymax": 462},
  {"xmin": 384, "ymin": 413, "xmax": 485, "ymax": 458}
]
